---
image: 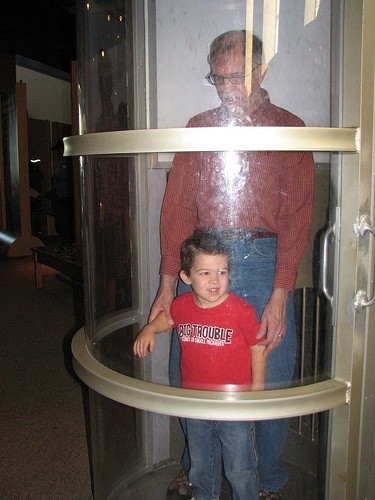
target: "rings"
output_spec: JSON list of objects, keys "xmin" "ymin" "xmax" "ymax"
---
[{"xmin": 277, "ymin": 334, "xmax": 284, "ymax": 340}]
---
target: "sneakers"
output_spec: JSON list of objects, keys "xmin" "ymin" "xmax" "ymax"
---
[
  {"xmin": 166, "ymin": 469, "xmax": 194, "ymax": 500},
  {"xmin": 257, "ymin": 488, "xmax": 282, "ymax": 500}
]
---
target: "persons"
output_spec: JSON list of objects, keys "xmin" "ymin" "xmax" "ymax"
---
[
  {"xmin": 147, "ymin": 29, "xmax": 320, "ymax": 500},
  {"xmin": 132, "ymin": 230, "xmax": 268, "ymax": 498}
]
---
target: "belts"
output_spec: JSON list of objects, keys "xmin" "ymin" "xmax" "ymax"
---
[{"xmin": 194, "ymin": 229, "xmax": 276, "ymax": 240}]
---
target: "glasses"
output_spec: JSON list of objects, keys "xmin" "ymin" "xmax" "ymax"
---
[{"xmin": 205, "ymin": 63, "xmax": 260, "ymax": 85}]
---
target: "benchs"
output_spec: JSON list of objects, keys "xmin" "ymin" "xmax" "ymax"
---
[{"xmin": 29, "ymin": 238, "xmax": 111, "ymax": 324}]
